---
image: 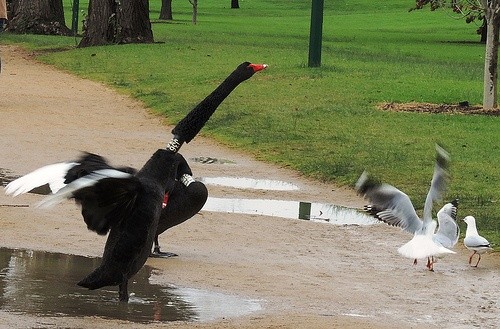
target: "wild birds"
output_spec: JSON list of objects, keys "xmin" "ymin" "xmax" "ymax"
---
[
  {"xmin": 353, "ymin": 141, "xmax": 460, "ymax": 272},
  {"xmin": 461, "ymin": 215, "xmax": 494, "ymax": 267}
]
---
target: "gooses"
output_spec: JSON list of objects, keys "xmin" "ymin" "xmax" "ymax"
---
[{"xmin": 2, "ymin": 61, "xmax": 268, "ymax": 302}]
